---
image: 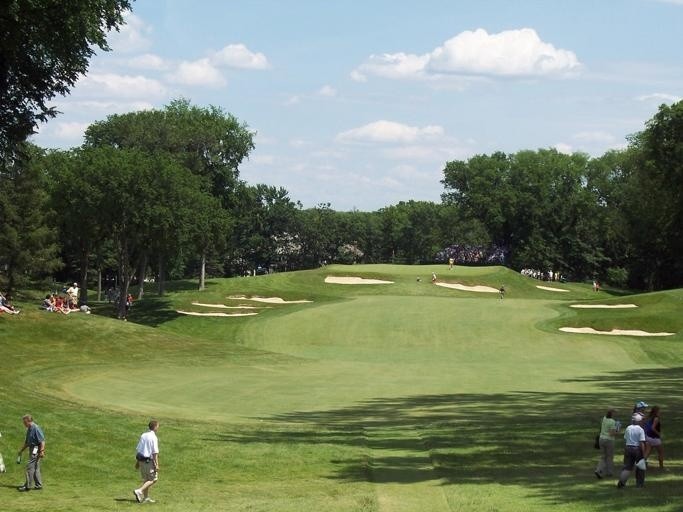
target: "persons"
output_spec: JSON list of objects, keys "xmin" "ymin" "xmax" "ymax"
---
[
  {"xmin": 16, "ymin": 414, "xmax": 47, "ymax": 493},
  {"xmin": 0, "ymin": 294, "xmax": 20, "ymax": 316},
  {"xmin": 104, "ymin": 286, "xmax": 133, "ymax": 320},
  {"xmin": 498, "ymin": 284, "xmax": 507, "ymax": 301},
  {"xmin": 41, "ymin": 282, "xmax": 92, "ymax": 317},
  {"xmin": 592, "ymin": 280, "xmax": 600, "ymax": 294},
  {"xmin": 592, "ymin": 401, "xmax": 663, "ymax": 488},
  {"xmin": 432, "ymin": 241, "xmax": 506, "ymax": 270},
  {"xmin": 516, "ymin": 266, "xmax": 567, "ymax": 285},
  {"xmin": 430, "ymin": 270, "xmax": 437, "ymax": 287},
  {"xmin": 132, "ymin": 421, "xmax": 161, "ymax": 504}
]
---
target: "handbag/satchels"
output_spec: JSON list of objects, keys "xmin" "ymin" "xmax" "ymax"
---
[{"xmin": 594, "ymin": 434, "xmax": 600, "ymax": 450}]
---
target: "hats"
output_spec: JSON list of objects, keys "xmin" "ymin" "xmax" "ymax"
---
[
  {"xmin": 631, "ymin": 412, "xmax": 642, "ymax": 421},
  {"xmin": 634, "ymin": 401, "xmax": 649, "ymax": 407}
]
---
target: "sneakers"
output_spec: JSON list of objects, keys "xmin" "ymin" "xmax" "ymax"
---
[
  {"xmin": 593, "ymin": 470, "xmax": 604, "ymax": 479},
  {"xmin": 142, "ymin": 496, "xmax": 155, "ymax": 503},
  {"xmin": 131, "ymin": 488, "xmax": 142, "ymax": 503}
]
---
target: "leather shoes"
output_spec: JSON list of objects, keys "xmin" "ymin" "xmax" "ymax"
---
[
  {"xmin": 15, "ymin": 485, "xmax": 30, "ymax": 490},
  {"xmin": 32, "ymin": 484, "xmax": 42, "ymax": 489}
]
